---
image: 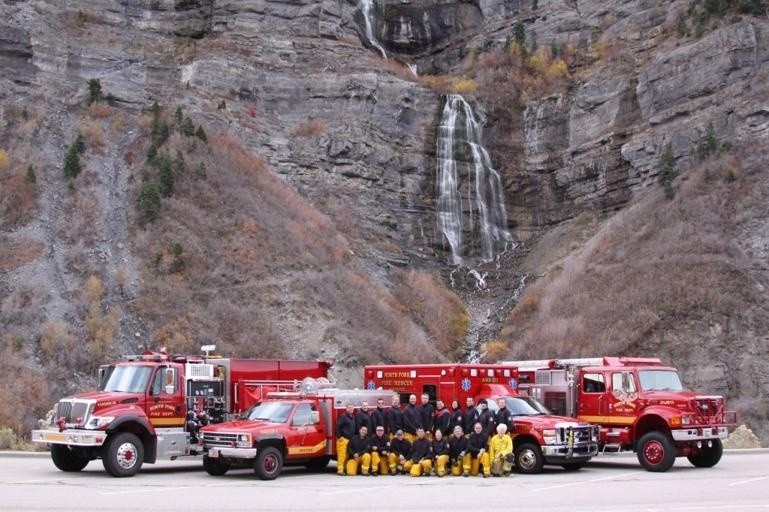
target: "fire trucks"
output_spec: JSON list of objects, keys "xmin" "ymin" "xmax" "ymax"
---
[
  {"xmin": 198, "ymin": 387, "xmax": 399, "ymax": 478},
  {"xmin": 32, "ymin": 346, "xmax": 333, "ymax": 478},
  {"xmin": 497, "ymin": 356, "xmax": 738, "ymax": 472},
  {"xmin": 364, "ymin": 364, "xmax": 600, "ymax": 474}
]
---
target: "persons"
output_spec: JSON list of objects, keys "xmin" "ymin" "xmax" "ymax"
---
[
  {"xmin": 389, "ymin": 428, "xmax": 413, "ymax": 476},
  {"xmin": 432, "ymin": 428, "xmax": 451, "ymax": 477},
  {"xmin": 335, "ymin": 403, "xmax": 355, "ymax": 476},
  {"xmin": 479, "ymin": 399, "xmax": 495, "ymax": 437},
  {"xmin": 408, "ymin": 425, "xmax": 434, "ymax": 477},
  {"xmin": 401, "ymin": 393, "xmax": 423, "ymax": 444},
  {"xmin": 417, "ymin": 393, "xmax": 435, "ymax": 433},
  {"xmin": 495, "ymin": 397, "xmax": 514, "ymax": 435},
  {"xmin": 345, "ymin": 424, "xmax": 372, "ymax": 476},
  {"xmin": 387, "ymin": 392, "xmax": 405, "ymax": 441},
  {"xmin": 450, "ymin": 424, "xmax": 472, "ymax": 477},
  {"xmin": 371, "ymin": 397, "xmax": 387, "ymax": 435},
  {"xmin": 449, "ymin": 400, "xmax": 465, "ymax": 438},
  {"xmin": 370, "ymin": 424, "xmax": 391, "ymax": 477},
  {"xmin": 433, "ymin": 399, "xmax": 451, "ymax": 438},
  {"xmin": 464, "ymin": 395, "xmax": 480, "ymax": 433},
  {"xmin": 356, "ymin": 400, "xmax": 373, "ymax": 436},
  {"xmin": 466, "ymin": 422, "xmax": 491, "ymax": 478},
  {"xmin": 489, "ymin": 423, "xmax": 514, "ymax": 477}
]
